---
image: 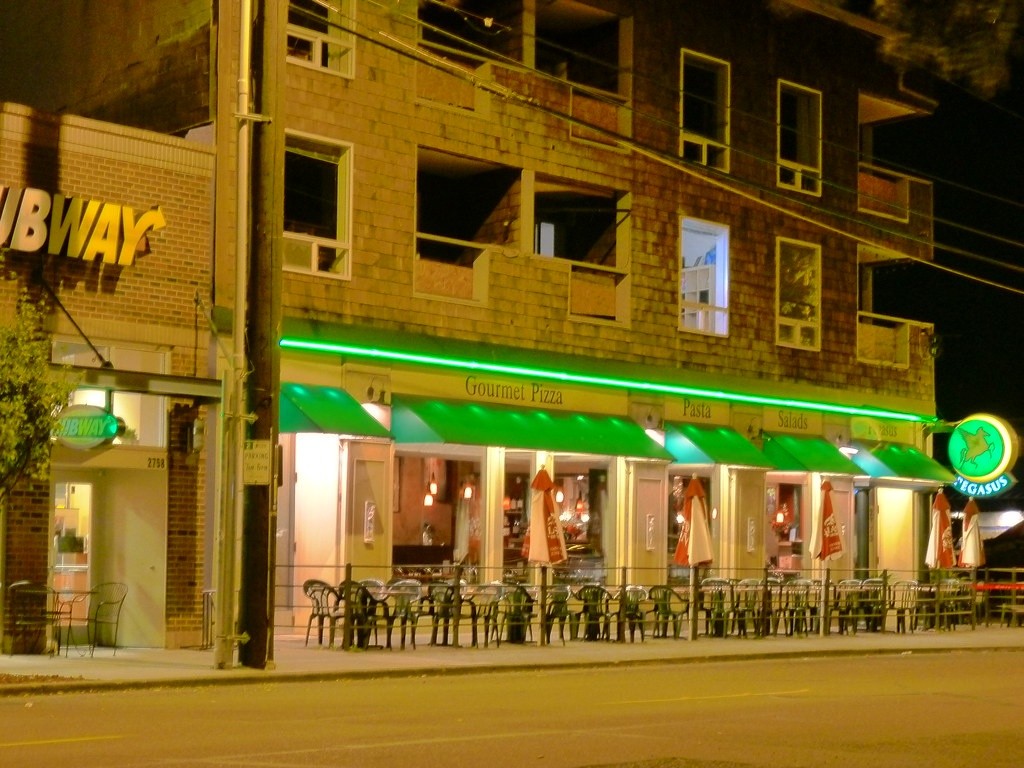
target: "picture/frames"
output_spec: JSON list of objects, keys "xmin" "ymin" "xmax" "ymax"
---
[{"xmin": 394, "ymin": 456, "xmax": 401, "ymax": 514}]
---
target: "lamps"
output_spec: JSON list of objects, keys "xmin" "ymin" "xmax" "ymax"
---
[
  {"xmin": 646, "ymin": 407, "xmax": 664, "ymax": 429},
  {"xmin": 367, "ymin": 375, "xmax": 387, "ymax": 405},
  {"xmin": 424, "ymin": 483, "xmax": 434, "ymax": 506},
  {"xmin": 429, "ymin": 471, "xmax": 438, "ymax": 494},
  {"xmin": 747, "ymin": 417, "xmax": 763, "ymax": 437},
  {"xmin": 837, "ymin": 426, "xmax": 855, "ymax": 447}
]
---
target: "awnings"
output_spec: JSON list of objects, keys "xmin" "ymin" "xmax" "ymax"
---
[
  {"xmin": 852, "ymin": 439, "xmax": 958, "ymax": 483},
  {"xmin": 762, "ymin": 430, "xmax": 868, "ymax": 477},
  {"xmin": 278, "ymin": 382, "xmax": 396, "ymax": 442},
  {"xmin": 664, "ymin": 419, "xmax": 779, "ymax": 470},
  {"xmin": 389, "ymin": 390, "xmax": 678, "ymax": 462}
]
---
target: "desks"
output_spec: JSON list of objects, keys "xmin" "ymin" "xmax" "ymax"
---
[
  {"xmin": 309, "ymin": 586, "xmax": 420, "ymax": 649},
  {"xmin": 829, "ymin": 587, "xmax": 866, "ymax": 635},
  {"xmin": 909, "ymin": 587, "xmax": 960, "ymax": 632},
  {"xmin": 601, "ymin": 597, "xmax": 654, "ymax": 643},
  {"xmin": 460, "ymin": 591, "xmax": 498, "ymax": 647},
  {"xmin": 506, "ymin": 588, "xmax": 567, "ymax": 645},
  {"xmin": 16, "ymin": 588, "xmax": 98, "ymax": 657}
]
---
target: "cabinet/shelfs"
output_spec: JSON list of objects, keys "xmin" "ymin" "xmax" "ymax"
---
[{"xmin": 681, "ymin": 264, "xmax": 716, "ymax": 331}]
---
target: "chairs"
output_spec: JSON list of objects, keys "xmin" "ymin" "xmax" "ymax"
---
[
  {"xmin": 998, "ymin": 578, "xmax": 1020, "ymax": 629},
  {"xmin": 65, "ymin": 582, "xmax": 128, "ymax": 658},
  {"xmin": 302, "ymin": 576, "xmax": 993, "ymax": 652},
  {"xmin": 7, "ymin": 580, "xmax": 61, "ymax": 660}
]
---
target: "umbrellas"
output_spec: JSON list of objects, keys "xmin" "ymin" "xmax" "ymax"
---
[
  {"xmin": 521, "ymin": 465, "xmax": 567, "ymax": 647},
  {"xmin": 924, "ymin": 487, "xmax": 957, "ymax": 570},
  {"xmin": 453, "ymin": 473, "xmax": 480, "ymax": 582},
  {"xmin": 808, "ymin": 478, "xmax": 843, "ymax": 638},
  {"xmin": 958, "ymin": 495, "xmax": 986, "ymax": 568},
  {"xmin": 674, "ymin": 473, "xmax": 714, "ymax": 641}
]
